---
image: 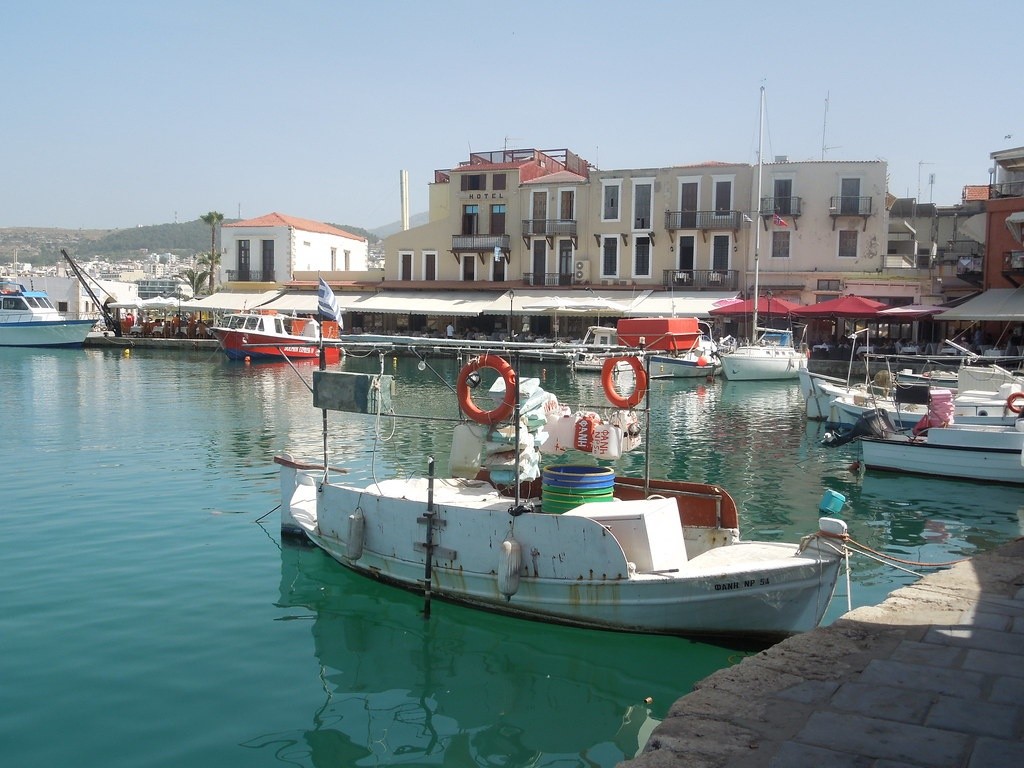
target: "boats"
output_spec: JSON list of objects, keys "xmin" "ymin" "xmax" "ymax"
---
[
  {"xmin": 275, "ymin": 273, "xmax": 852, "ymax": 642},
  {"xmin": 0, "ymin": 277, "xmax": 103, "ymax": 347},
  {"xmin": 563, "ymin": 279, "xmax": 723, "ymax": 381},
  {"xmin": 791, "ymin": 327, "xmax": 1024, "ymax": 487},
  {"xmin": 208, "ymin": 292, "xmax": 343, "ymax": 359}
]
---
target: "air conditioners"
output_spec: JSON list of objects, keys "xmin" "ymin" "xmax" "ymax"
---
[
  {"xmin": 574, "ymin": 261, "xmax": 589, "ymax": 282},
  {"xmin": 601, "ymin": 279, "xmax": 614, "ymax": 285},
  {"xmin": 619, "ymin": 280, "xmax": 632, "ymax": 285}
]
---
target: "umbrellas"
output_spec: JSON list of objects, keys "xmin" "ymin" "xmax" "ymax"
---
[
  {"xmin": 107, "ymin": 296, "xmax": 184, "ymax": 311},
  {"xmin": 707, "ymin": 294, "xmax": 948, "ymax": 355}
]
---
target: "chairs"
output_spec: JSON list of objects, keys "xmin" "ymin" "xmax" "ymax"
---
[
  {"xmin": 351, "ymin": 326, "xmax": 548, "ymax": 342},
  {"xmin": 118, "ymin": 320, "xmax": 208, "ymax": 339}
]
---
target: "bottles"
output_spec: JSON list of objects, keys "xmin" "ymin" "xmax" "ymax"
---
[{"xmin": 448, "ymin": 424, "xmax": 483, "ymax": 479}]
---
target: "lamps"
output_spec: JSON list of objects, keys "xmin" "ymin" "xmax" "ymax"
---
[{"xmin": 987, "ymin": 168, "xmax": 995, "ymax": 185}]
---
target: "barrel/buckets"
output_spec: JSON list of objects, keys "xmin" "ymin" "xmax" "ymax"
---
[
  {"xmin": 541, "ymin": 409, "xmax": 643, "ymax": 460},
  {"xmin": 818, "ymin": 489, "xmax": 846, "ymax": 513},
  {"xmin": 540, "ymin": 464, "xmax": 616, "ymax": 513}
]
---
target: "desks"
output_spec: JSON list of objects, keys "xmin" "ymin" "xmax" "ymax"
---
[
  {"xmin": 674, "ymin": 273, "xmax": 689, "ymax": 286},
  {"xmin": 195, "ymin": 326, "xmax": 211, "ymax": 338},
  {"xmin": 151, "ymin": 326, "xmax": 170, "ymax": 338},
  {"xmin": 175, "ymin": 327, "xmax": 188, "ymax": 338},
  {"xmin": 707, "ymin": 271, "xmax": 722, "ymax": 286},
  {"xmin": 129, "ymin": 326, "xmax": 144, "ymax": 338}
]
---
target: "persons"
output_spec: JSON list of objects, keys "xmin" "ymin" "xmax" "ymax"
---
[
  {"xmin": 815, "ymin": 331, "xmax": 951, "ymax": 356},
  {"xmin": 974, "ymin": 326, "xmax": 982, "ymax": 345},
  {"xmin": 462, "ymin": 327, "xmax": 474, "ymax": 340},
  {"xmin": 1004, "ymin": 324, "xmax": 1022, "ymax": 347},
  {"xmin": 136, "ymin": 313, "xmax": 143, "ymax": 326},
  {"xmin": 145, "ymin": 313, "xmax": 152, "ymax": 322},
  {"xmin": 130, "ymin": 314, "xmax": 134, "ymax": 325},
  {"xmin": 171, "ymin": 314, "xmax": 179, "ymax": 328},
  {"xmin": 961, "ymin": 337, "xmax": 969, "ymax": 345},
  {"xmin": 444, "ymin": 322, "xmax": 454, "ymax": 338}
]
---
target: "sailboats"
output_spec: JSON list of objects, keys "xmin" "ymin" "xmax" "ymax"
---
[{"xmin": 713, "ymin": 85, "xmax": 812, "ymax": 383}]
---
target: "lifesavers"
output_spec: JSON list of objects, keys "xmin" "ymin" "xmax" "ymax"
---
[
  {"xmin": 126, "ymin": 313, "xmax": 134, "ymax": 322},
  {"xmin": 457, "ymin": 354, "xmax": 516, "ymax": 425},
  {"xmin": 1008, "ymin": 393, "xmax": 1024, "ymax": 413},
  {"xmin": 601, "ymin": 356, "xmax": 648, "ymax": 409}
]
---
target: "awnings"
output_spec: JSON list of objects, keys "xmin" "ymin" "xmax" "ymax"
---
[
  {"xmin": 186, "ymin": 291, "xmax": 377, "ymax": 316},
  {"xmin": 346, "ymin": 291, "xmax": 505, "ymax": 317},
  {"xmin": 483, "ymin": 288, "xmax": 741, "ymax": 318},
  {"xmin": 932, "ymin": 289, "xmax": 1024, "ymax": 321}
]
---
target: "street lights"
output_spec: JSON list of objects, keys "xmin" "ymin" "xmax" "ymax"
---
[
  {"xmin": 765, "ymin": 288, "xmax": 774, "ymax": 329},
  {"xmin": 176, "ymin": 286, "xmax": 183, "ymax": 338},
  {"xmin": 508, "ymin": 289, "xmax": 515, "ymax": 343}
]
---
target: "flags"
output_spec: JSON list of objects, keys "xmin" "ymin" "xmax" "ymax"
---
[
  {"xmin": 318, "ymin": 278, "xmax": 343, "ymax": 329},
  {"xmin": 773, "ymin": 214, "xmax": 787, "ymax": 226}
]
